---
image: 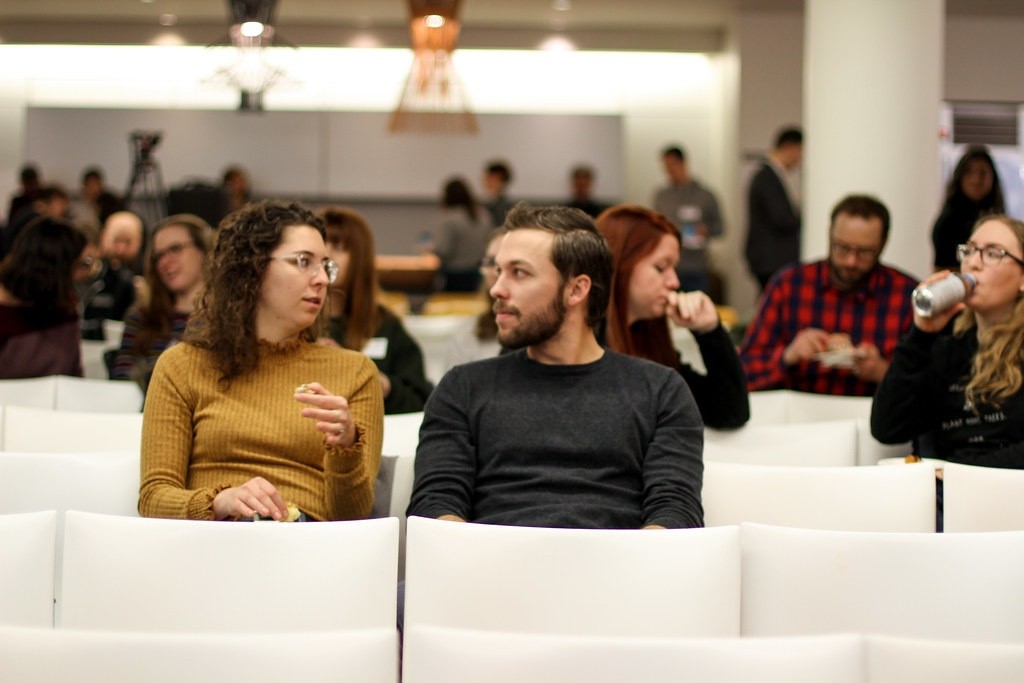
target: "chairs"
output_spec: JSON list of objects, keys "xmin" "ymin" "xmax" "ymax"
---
[{"xmin": 0, "ymin": 296, "xmax": 1023, "ymax": 683}]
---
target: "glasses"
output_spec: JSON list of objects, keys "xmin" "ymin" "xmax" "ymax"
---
[
  {"xmin": 151, "ymin": 239, "xmax": 196, "ymax": 268},
  {"xmin": 265, "ymin": 254, "xmax": 340, "ymax": 286},
  {"xmin": 956, "ymin": 243, "xmax": 1024, "ymax": 270},
  {"xmin": 832, "ymin": 239, "xmax": 882, "ymax": 263}
]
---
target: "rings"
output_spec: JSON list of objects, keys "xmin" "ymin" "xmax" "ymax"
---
[{"xmin": 337, "ymin": 429, "xmax": 345, "ymax": 433}]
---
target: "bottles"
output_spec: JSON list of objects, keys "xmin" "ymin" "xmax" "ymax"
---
[{"xmin": 911, "ymin": 272, "xmax": 978, "ymax": 319}]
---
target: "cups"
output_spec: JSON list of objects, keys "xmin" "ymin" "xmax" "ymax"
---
[{"xmin": 676, "ymin": 206, "xmax": 703, "ymax": 250}]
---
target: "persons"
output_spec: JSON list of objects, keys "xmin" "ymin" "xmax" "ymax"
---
[
  {"xmin": 138, "ymin": 199, "xmax": 384, "ymax": 521},
  {"xmin": 562, "ymin": 166, "xmax": 609, "ymax": 219},
  {"xmin": 653, "ymin": 148, "xmax": 723, "ymax": 292},
  {"xmin": 308, "ymin": 210, "xmax": 434, "ymax": 415},
  {"xmin": 482, "ymin": 164, "xmax": 516, "ymax": 226},
  {"xmin": 112, "ymin": 215, "xmax": 214, "ymax": 412},
  {"xmin": 431, "ymin": 164, "xmax": 496, "ymax": 294},
  {"xmin": 406, "ymin": 201, "xmax": 704, "ymax": 529},
  {"xmin": 742, "ymin": 129, "xmax": 803, "ymax": 294},
  {"xmin": 870, "ymin": 214, "xmax": 1024, "ymax": 470},
  {"xmin": 932, "ymin": 145, "xmax": 1006, "ymax": 275},
  {"xmin": 739, "ymin": 195, "xmax": 922, "ymax": 398},
  {"xmin": 0, "ymin": 168, "xmax": 251, "ymax": 380},
  {"xmin": 595, "ymin": 204, "xmax": 750, "ymax": 431}
]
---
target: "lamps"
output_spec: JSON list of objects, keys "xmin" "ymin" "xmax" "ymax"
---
[
  {"xmin": 383, "ymin": 0, "xmax": 484, "ymax": 142},
  {"xmin": 220, "ymin": 0, "xmax": 284, "ymax": 116}
]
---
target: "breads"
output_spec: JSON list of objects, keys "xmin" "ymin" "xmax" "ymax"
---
[
  {"xmin": 825, "ymin": 333, "xmax": 854, "ymax": 351},
  {"xmin": 905, "ymin": 454, "xmax": 923, "ymax": 464},
  {"xmin": 295, "ymin": 384, "xmax": 317, "ymax": 393},
  {"xmin": 279, "ymin": 502, "xmax": 300, "ymax": 522}
]
